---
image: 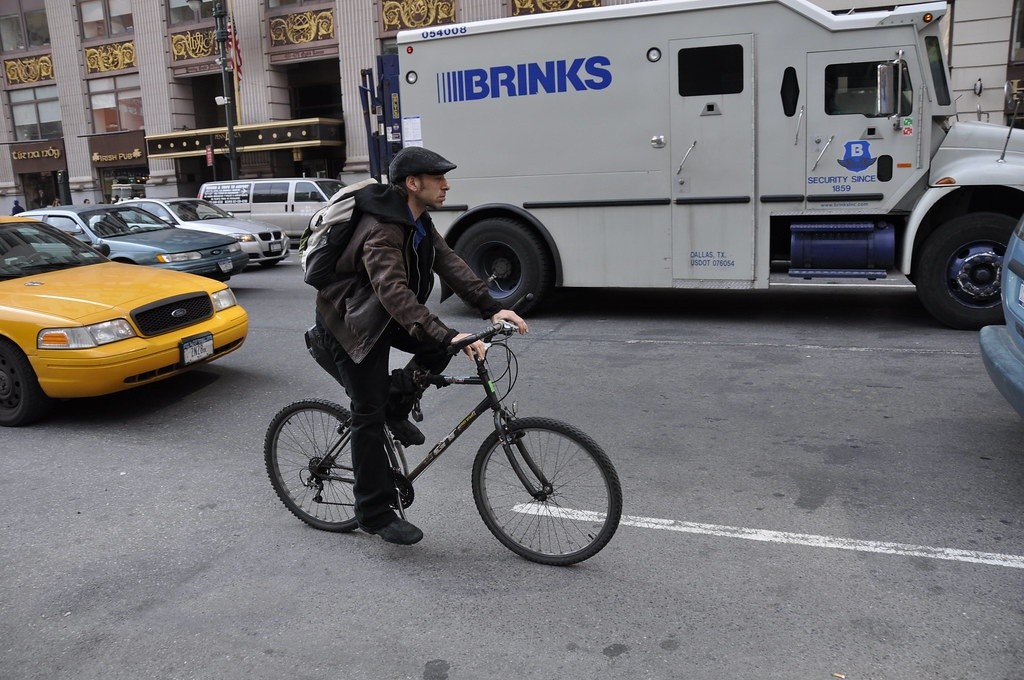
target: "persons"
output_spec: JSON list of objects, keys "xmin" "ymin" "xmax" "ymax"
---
[
  {"xmin": 314, "ymin": 147, "xmax": 531, "ymax": 544},
  {"xmin": 52, "ymin": 199, "xmax": 61, "ymax": 207},
  {"xmin": 12, "ymin": 200, "xmax": 25, "ymax": 216},
  {"xmin": 84, "ymin": 199, "xmax": 90, "ymax": 204},
  {"xmin": 112, "ymin": 195, "xmax": 123, "ymax": 205}
]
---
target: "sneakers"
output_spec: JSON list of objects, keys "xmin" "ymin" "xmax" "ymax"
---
[
  {"xmin": 384, "ymin": 409, "xmax": 426, "ymax": 446},
  {"xmin": 357, "ymin": 504, "xmax": 424, "ymax": 544}
]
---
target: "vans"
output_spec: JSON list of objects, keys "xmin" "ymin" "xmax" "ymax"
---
[{"xmin": 197, "ymin": 178, "xmax": 349, "ymax": 245}]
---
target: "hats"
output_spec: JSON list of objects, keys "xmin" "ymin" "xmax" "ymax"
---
[{"xmin": 388, "ymin": 147, "xmax": 458, "ymax": 182}]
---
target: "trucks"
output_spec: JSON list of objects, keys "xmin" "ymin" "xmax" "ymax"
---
[{"xmin": 357, "ymin": 1, "xmax": 1024, "ymax": 330}]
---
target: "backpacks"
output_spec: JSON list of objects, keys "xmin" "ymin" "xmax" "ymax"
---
[{"xmin": 299, "ymin": 177, "xmax": 382, "ymax": 292}]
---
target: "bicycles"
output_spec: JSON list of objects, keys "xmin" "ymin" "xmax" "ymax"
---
[{"xmin": 262, "ymin": 318, "xmax": 625, "ymax": 567}]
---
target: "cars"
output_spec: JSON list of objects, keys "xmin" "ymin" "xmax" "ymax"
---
[
  {"xmin": 113, "ymin": 196, "xmax": 291, "ymax": 267},
  {"xmin": 0, "ymin": 215, "xmax": 251, "ymax": 427},
  {"xmin": 13, "ymin": 203, "xmax": 250, "ymax": 283}
]
